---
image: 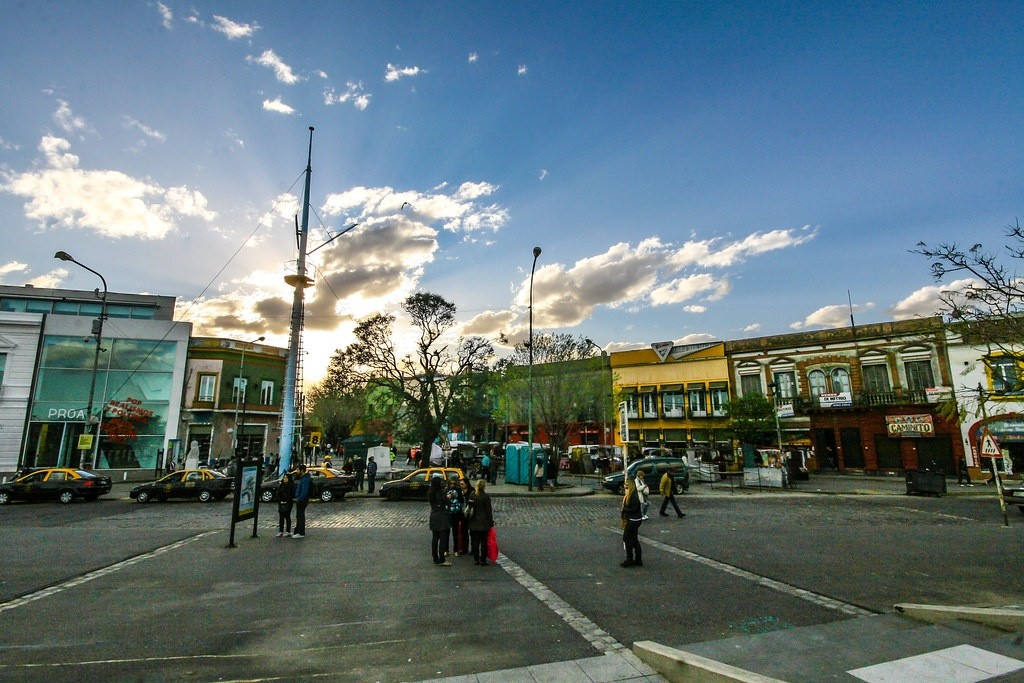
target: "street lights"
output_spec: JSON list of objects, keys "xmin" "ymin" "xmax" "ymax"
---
[
  {"xmin": 586, "ymin": 339, "xmax": 607, "ymax": 445},
  {"xmin": 768, "ymin": 380, "xmax": 787, "ymax": 485}
]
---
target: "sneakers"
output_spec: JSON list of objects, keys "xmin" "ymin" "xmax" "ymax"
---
[
  {"xmin": 291, "ymin": 534, "xmax": 305, "ymax": 538},
  {"xmin": 282, "ymin": 532, "xmax": 291, "ymax": 537},
  {"xmin": 276, "ymin": 532, "xmax": 283, "ymax": 537}
]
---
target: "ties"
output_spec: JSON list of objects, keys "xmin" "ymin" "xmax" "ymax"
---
[{"xmin": 171, "ymin": 463, "xmax": 173, "ymax": 469}]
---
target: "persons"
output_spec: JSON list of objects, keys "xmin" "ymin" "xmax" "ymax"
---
[
  {"xmin": 534, "ymin": 458, "xmax": 556, "ymax": 492},
  {"xmin": 659, "ymin": 467, "xmax": 686, "ymax": 518},
  {"xmin": 390, "ymin": 447, "xmax": 421, "ymax": 468},
  {"xmin": 166, "ymin": 453, "xmax": 278, "ymax": 477},
  {"xmin": 958, "ymin": 456, "xmax": 973, "ymax": 487},
  {"xmin": 597, "ymin": 448, "xmax": 645, "ymax": 473},
  {"xmin": 305, "ymin": 442, "xmax": 343, "ymax": 464},
  {"xmin": 322, "ymin": 455, "xmax": 377, "ymax": 493},
  {"xmin": 427, "ymin": 475, "xmax": 494, "ymax": 565},
  {"xmin": 985, "ymin": 459, "xmax": 1001, "ymax": 485},
  {"xmin": 451, "ymin": 453, "xmax": 503, "ymax": 485},
  {"xmin": 619, "ymin": 478, "xmax": 643, "ymax": 567},
  {"xmin": 275, "ymin": 465, "xmax": 311, "ymax": 539},
  {"xmin": 635, "ymin": 471, "xmax": 649, "ymax": 519}
]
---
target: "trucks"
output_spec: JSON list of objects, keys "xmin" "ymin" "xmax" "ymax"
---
[
  {"xmin": 449, "ymin": 441, "xmax": 549, "ymax": 460},
  {"xmin": 568, "ymin": 445, "xmax": 621, "ymax": 466}
]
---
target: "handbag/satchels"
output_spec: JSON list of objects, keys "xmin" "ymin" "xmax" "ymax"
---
[
  {"xmin": 446, "ymin": 490, "xmax": 461, "ymax": 511},
  {"xmin": 620, "ymin": 496, "xmax": 627, "ymax": 519},
  {"xmin": 488, "ymin": 528, "xmax": 498, "ymax": 561},
  {"xmin": 464, "ymin": 505, "xmax": 470, "ymax": 517},
  {"xmin": 643, "ymin": 487, "xmax": 650, "ymax": 495}
]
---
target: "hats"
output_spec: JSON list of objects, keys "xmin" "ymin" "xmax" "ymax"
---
[
  {"xmin": 325, "ymin": 455, "xmax": 332, "ymax": 459},
  {"xmin": 637, "ymin": 471, "xmax": 644, "ymax": 476}
]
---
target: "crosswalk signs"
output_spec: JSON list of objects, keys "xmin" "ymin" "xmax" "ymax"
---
[{"xmin": 979, "ymin": 426, "xmax": 1004, "ymax": 458}]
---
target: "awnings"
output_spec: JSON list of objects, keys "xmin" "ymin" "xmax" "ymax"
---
[
  {"xmin": 620, "ymin": 388, "xmax": 636, "ymax": 395},
  {"xmin": 659, "ymin": 385, "xmax": 682, "ymax": 392},
  {"xmin": 638, "ymin": 387, "xmax": 656, "ymax": 394},
  {"xmin": 775, "ymin": 438, "xmax": 811, "ymax": 446},
  {"xmin": 686, "ymin": 382, "xmax": 727, "ymax": 391}
]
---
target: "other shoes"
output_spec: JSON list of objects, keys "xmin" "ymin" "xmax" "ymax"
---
[
  {"xmin": 642, "ymin": 517, "xmax": 646, "ymax": 520},
  {"xmin": 644, "ymin": 515, "xmax": 649, "ymax": 519},
  {"xmin": 966, "ymin": 484, "xmax": 974, "ymax": 487},
  {"xmin": 620, "ymin": 561, "xmax": 634, "ymax": 567},
  {"xmin": 958, "ymin": 484, "xmax": 964, "ymax": 487},
  {"xmin": 659, "ymin": 511, "xmax": 668, "ymax": 516},
  {"xmin": 434, "ymin": 550, "xmax": 487, "ymax": 565},
  {"xmin": 678, "ymin": 514, "xmax": 686, "ymax": 517},
  {"xmin": 985, "ymin": 480, "xmax": 989, "ymax": 486},
  {"xmin": 633, "ymin": 560, "xmax": 642, "ymax": 566}
]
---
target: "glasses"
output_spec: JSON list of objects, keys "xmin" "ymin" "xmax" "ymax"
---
[{"xmin": 449, "ymin": 482, "xmax": 456, "ymax": 485}]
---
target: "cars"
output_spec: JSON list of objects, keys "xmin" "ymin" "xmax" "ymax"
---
[
  {"xmin": 0, "ymin": 468, "xmax": 112, "ymax": 504},
  {"xmin": 378, "ymin": 466, "xmax": 468, "ymax": 500},
  {"xmin": 528, "ymin": 247, "xmax": 542, "ymax": 493},
  {"xmin": 257, "ymin": 467, "xmax": 356, "ymax": 504},
  {"xmin": 129, "ymin": 467, "xmax": 235, "ymax": 503},
  {"xmin": 233, "ymin": 337, "xmax": 266, "ymax": 455},
  {"xmin": 55, "ymin": 249, "xmax": 108, "ymax": 470}
]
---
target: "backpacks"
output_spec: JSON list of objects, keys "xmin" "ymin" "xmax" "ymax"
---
[{"xmin": 309, "ymin": 477, "xmax": 318, "ymax": 498}]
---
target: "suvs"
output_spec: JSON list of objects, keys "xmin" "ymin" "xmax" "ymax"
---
[{"xmin": 599, "ymin": 456, "xmax": 690, "ymax": 495}]
